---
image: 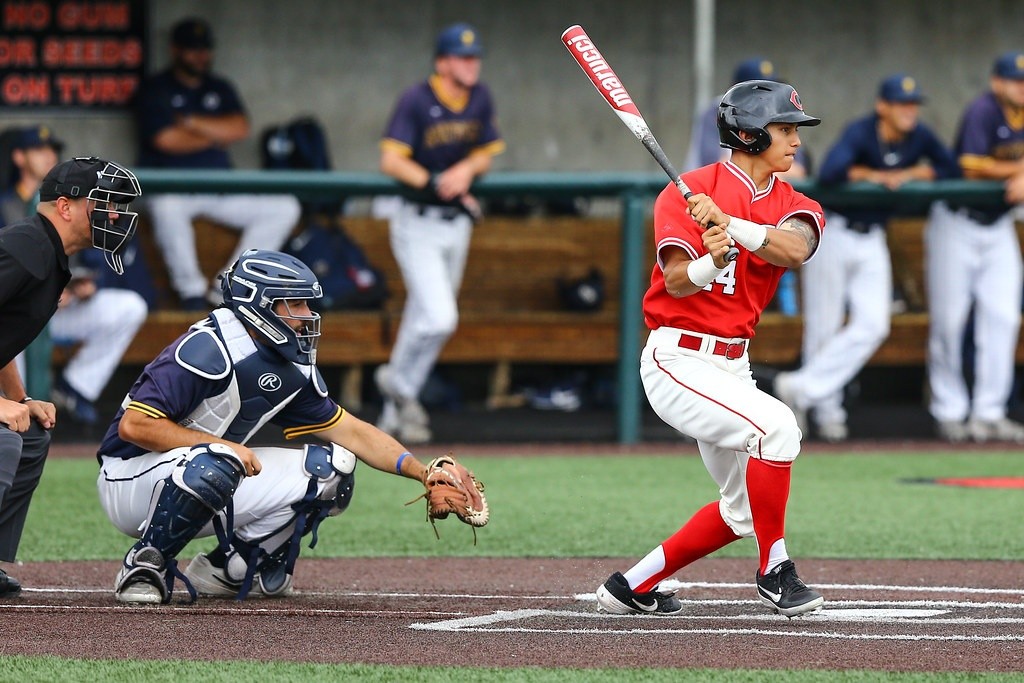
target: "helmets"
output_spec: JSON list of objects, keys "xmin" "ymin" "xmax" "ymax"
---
[
  {"xmin": 222, "ymin": 250, "xmax": 323, "ymax": 366},
  {"xmin": 718, "ymin": 80, "xmax": 821, "ymax": 155}
]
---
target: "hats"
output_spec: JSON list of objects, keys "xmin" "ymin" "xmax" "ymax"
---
[
  {"xmin": 995, "ymin": 52, "xmax": 1024, "ymax": 78},
  {"xmin": 733, "ymin": 57, "xmax": 787, "ymax": 84},
  {"xmin": 435, "ymin": 23, "xmax": 482, "ymax": 55},
  {"xmin": 9, "ymin": 125, "xmax": 63, "ymax": 151},
  {"xmin": 169, "ymin": 16, "xmax": 218, "ymax": 50},
  {"xmin": 40, "ymin": 156, "xmax": 107, "ymax": 203},
  {"xmin": 879, "ymin": 74, "xmax": 921, "ymax": 101}
]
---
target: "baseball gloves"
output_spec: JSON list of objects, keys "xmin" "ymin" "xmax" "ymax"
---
[{"xmin": 424, "ymin": 456, "xmax": 490, "ymax": 527}]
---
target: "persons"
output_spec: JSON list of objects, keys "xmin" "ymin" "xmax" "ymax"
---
[
  {"xmin": 595, "ymin": 80, "xmax": 824, "ymax": 618},
  {"xmin": 0, "ymin": 156, "xmax": 142, "ymax": 598},
  {"xmin": 127, "ymin": 16, "xmax": 304, "ymax": 310},
  {"xmin": 371, "ymin": 21, "xmax": 510, "ymax": 439},
  {"xmin": 0, "ymin": 124, "xmax": 70, "ymax": 227},
  {"xmin": 94, "ymin": 248, "xmax": 489, "ymax": 603},
  {"xmin": 772, "ymin": 55, "xmax": 1024, "ymax": 442},
  {"xmin": 697, "ymin": 52, "xmax": 812, "ymax": 183}
]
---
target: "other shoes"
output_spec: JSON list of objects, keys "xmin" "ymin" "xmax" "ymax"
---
[
  {"xmin": 776, "ymin": 373, "xmax": 851, "ymax": 442},
  {"xmin": 938, "ymin": 412, "xmax": 1021, "ymax": 445}
]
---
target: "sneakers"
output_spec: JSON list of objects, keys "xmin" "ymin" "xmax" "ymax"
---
[
  {"xmin": 596, "ymin": 571, "xmax": 682, "ymax": 616},
  {"xmin": 0, "ymin": 567, "xmax": 22, "ymax": 596},
  {"xmin": 399, "ymin": 396, "xmax": 432, "ymax": 442},
  {"xmin": 50, "ymin": 379, "xmax": 97, "ymax": 420},
  {"xmin": 114, "ymin": 551, "xmax": 162, "ymax": 603},
  {"xmin": 756, "ymin": 560, "xmax": 824, "ymax": 616},
  {"xmin": 183, "ymin": 552, "xmax": 264, "ymax": 595}
]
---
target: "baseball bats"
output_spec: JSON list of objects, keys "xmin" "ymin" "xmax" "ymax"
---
[{"xmin": 560, "ymin": 25, "xmax": 740, "ymax": 263}]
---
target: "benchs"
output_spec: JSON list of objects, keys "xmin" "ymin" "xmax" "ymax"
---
[{"xmin": 23, "ymin": 207, "xmax": 1024, "ymax": 411}]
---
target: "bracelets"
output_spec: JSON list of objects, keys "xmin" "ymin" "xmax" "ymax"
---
[
  {"xmin": 396, "ymin": 452, "xmax": 412, "ymax": 475},
  {"xmin": 19, "ymin": 396, "xmax": 32, "ymax": 403}
]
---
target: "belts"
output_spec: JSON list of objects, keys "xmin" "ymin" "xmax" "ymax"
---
[{"xmin": 679, "ymin": 333, "xmax": 745, "ymax": 360}]
